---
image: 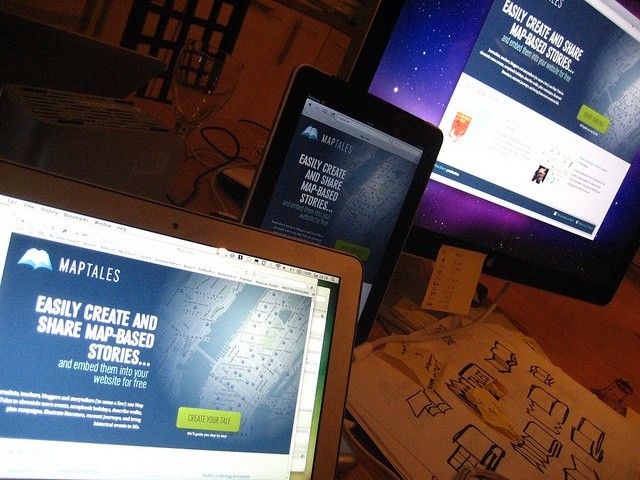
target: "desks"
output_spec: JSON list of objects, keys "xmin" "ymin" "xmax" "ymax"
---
[{"xmin": 0, "ymin": 93, "xmax": 640, "ymax": 480}]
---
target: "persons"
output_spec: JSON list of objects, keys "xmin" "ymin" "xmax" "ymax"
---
[{"xmin": 533, "ymin": 167, "xmax": 546, "ymax": 185}]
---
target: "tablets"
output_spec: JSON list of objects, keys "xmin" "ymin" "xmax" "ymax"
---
[{"xmin": 242, "ymin": 61, "xmax": 444, "ymax": 348}]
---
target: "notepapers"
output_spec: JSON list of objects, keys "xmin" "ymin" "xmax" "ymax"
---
[{"xmin": 392, "ymin": 296, "xmax": 439, "ymax": 330}]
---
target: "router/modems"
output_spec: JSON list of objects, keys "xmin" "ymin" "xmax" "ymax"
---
[{"xmin": 220, "ymin": 165, "xmax": 257, "ymax": 199}]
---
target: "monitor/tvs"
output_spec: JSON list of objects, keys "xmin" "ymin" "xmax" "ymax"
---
[
  {"xmin": 0, "ymin": 158, "xmax": 365, "ymax": 480},
  {"xmin": 338, "ymin": 0, "xmax": 639, "ymax": 306}
]
